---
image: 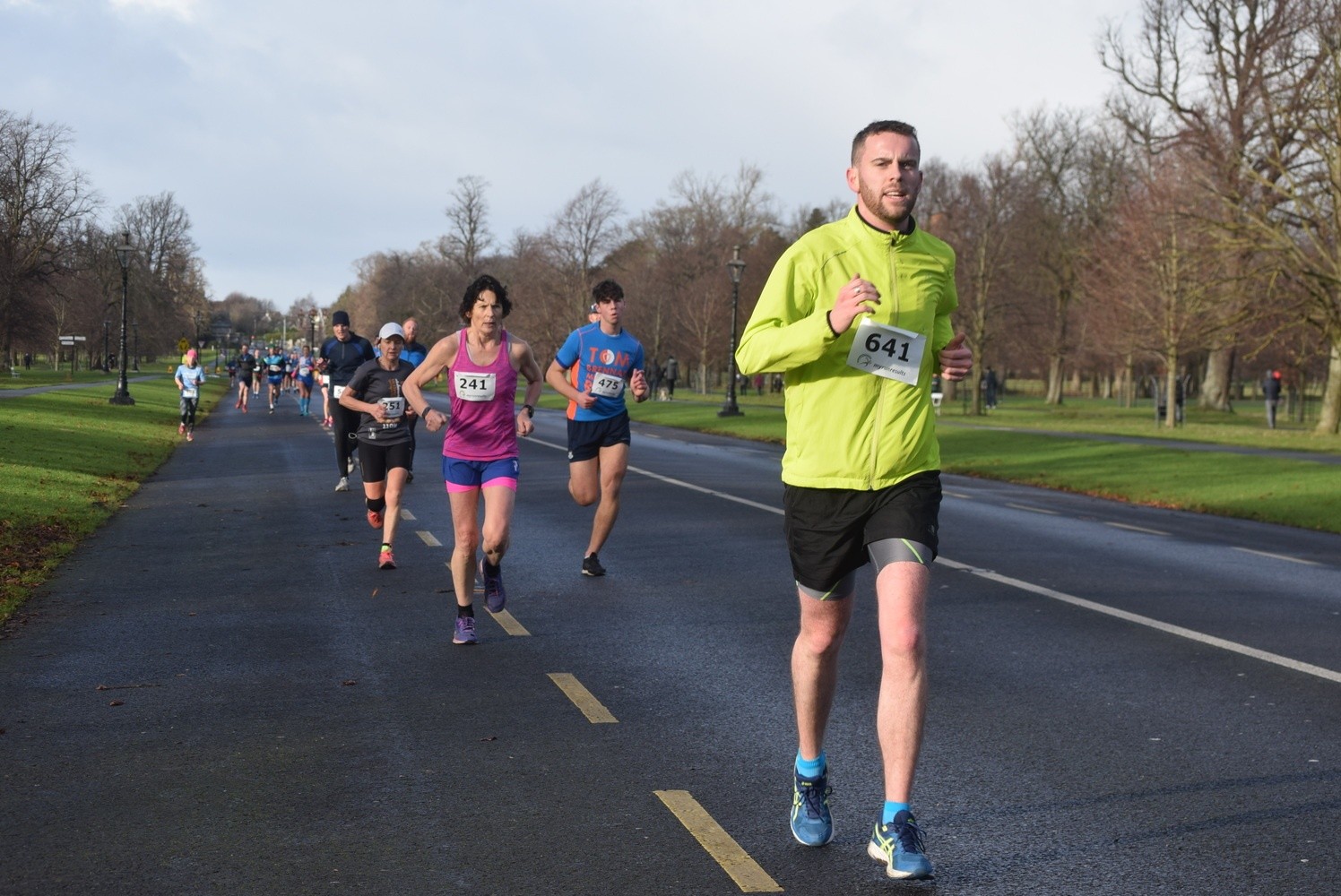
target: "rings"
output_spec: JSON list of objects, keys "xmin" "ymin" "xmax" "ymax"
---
[{"xmin": 855, "ymin": 287, "xmax": 859, "ymax": 292}]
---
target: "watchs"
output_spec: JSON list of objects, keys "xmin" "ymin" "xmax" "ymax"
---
[{"xmin": 521, "ymin": 404, "xmax": 534, "ymax": 419}]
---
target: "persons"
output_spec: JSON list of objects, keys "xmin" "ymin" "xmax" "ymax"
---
[
  {"xmin": 339, "ymin": 322, "xmax": 419, "ymax": 569},
  {"xmin": 734, "ymin": 121, "xmax": 972, "ymax": 878},
  {"xmin": 316, "ymin": 311, "xmax": 375, "ymax": 492},
  {"xmin": 754, "ymin": 373, "xmax": 763, "ymax": 396},
  {"xmin": 985, "ymin": 365, "xmax": 997, "ymax": 409},
  {"xmin": 401, "ymin": 275, "xmax": 544, "ymax": 644},
  {"xmin": 582, "ymin": 303, "xmax": 600, "ymax": 326},
  {"xmin": 107, "ymin": 352, "xmax": 115, "ymax": 370},
  {"xmin": 24, "ymin": 352, "xmax": 30, "ymax": 370},
  {"xmin": 737, "ymin": 375, "xmax": 749, "ymax": 396},
  {"xmin": 398, "ymin": 317, "xmax": 426, "ymax": 483},
  {"xmin": 1263, "ymin": 371, "xmax": 1283, "ymax": 429},
  {"xmin": 931, "ymin": 373, "xmax": 942, "ymax": 403},
  {"xmin": 545, "ymin": 280, "xmax": 649, "ymax": 577},
  {"xmin": 774, "ymin": 374, "xmax": 782, "ymax": 394},
  {"xmin": 225, "ymin": 343, "xmax": 335, "ymax": 428},
  {"xmin": 647, "ymin": 355, "xmax": 681, "ymax": 402},
  {"xmin": 174, "ymin": 350, "xmax": 206, "ymax": 442},
  {"xmin": 371, "ymin": 336, "xmax": 381, "ymax": 357},
  {"xmin": 1175, "ymin": 375, "xmax": 1186, "ymax": 423}
]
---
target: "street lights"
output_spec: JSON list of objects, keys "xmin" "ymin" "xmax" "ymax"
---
[
  {"xmin": 130, "ymin": 317, "xmax": 139, "ymax": 370},
  {"xmin": 193, "ymin": 310, "xmax": 203, "ymax": 361},
  {"xmin": 109, "ymin": 230, "xmax": 139, "ymax": 405},
  {"xmin": 718, "ymin": 245, "xmax": 747, "ymax": 417},
  {"xmin": 101, "ymin": 318, "xmax": 111, "ymax": 374}
]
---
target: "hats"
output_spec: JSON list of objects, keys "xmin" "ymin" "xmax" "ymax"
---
[
  {"xmin": 379, "ymin": 322, "xmax": 405, "ymax": 340},
  {"xmin": 332, "ymin": 311, "xmax": 349, "ymax": 326},
  {"xmin": 589, "ymin": 303, "xmax": 598, "ymax": 314}
]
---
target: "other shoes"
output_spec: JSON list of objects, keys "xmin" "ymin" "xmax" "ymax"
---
[
  {"xmin": 406, "ymin": 472, "xmax": 413, "ymax": 483},
  {"xmin": 328, "ymin": 419, "xmax": 335, "ymax": 428},
  {"xmin": 230, "ymin": 380, "xmax": 311, "ymax": 418},
  {"xmin": 322, "ymin": 419, "xmax": 329, "ymax": 426}
]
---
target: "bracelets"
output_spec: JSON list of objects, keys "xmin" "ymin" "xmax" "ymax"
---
[{"xmin": 422, "ymin": 407, "xmax": 431, "ymax": 420}]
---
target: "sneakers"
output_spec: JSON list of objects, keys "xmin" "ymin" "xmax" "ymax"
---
[
  {"xmin": 347, "ymin": 456, "xmax": 355, "ymax": 474},
  {"xmin": 365, "ymin": 496, "xmax": 384, "ymax": 529},
  {"xmin": 179, "ymin": 424, "xmax": 185, "ymax": 434},
  {"xmin": 867, "ymin": 810, "xmax": 933, "ymax": 880},
  {"xmin": 378, "ymin": 544, "xmax": 396, "ymax": 569},
  {"xmin": 335, "ymin": 477, "xmax": 349, "ymax": 491},
  {"xmin": 581, "ymin": 552, "xmax": 606, "ymax": 576},
  {"xmin": 789, "ymin": 753, "xmax": 834, "ymax": 847},
  {"xmin": 187, "ymin": 433, "xmax": 194, "ymax": 442},
  {"xmin": 242, "ymin": 405, "xmax": 247, "ymax": 413},
  {"xmin": 236, "ymin": 400, "xmax": 242, "ymax": 408},
  {"xmin": 479, "ymin": 555, "xmax": 505, "ymax": 613},
  {"xmin": 453, "ymin": 614, "xmax": 482, "ymax": 645}
]
